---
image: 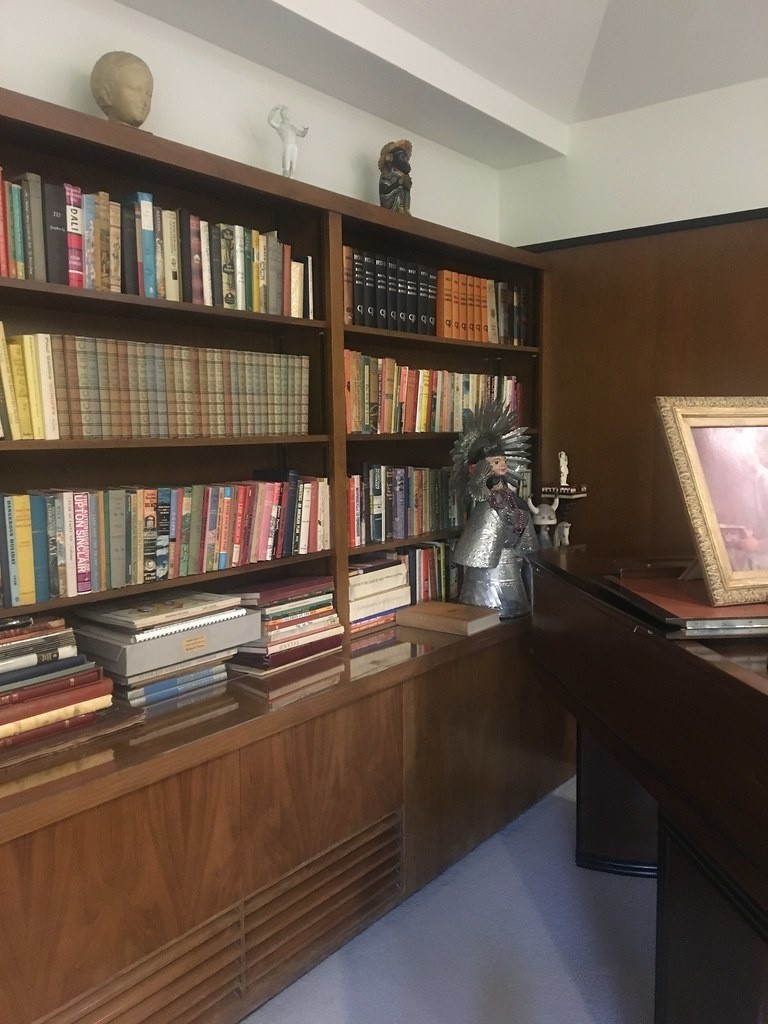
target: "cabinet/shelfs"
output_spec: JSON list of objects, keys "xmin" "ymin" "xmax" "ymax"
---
[{"xmin": 0, "ymin": 85, "xmax": 573, "ymax": 1024}]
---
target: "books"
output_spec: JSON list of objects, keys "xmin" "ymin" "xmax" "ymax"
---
[
  {"xmin": 348, "ymin": 462, "xmax": 477, "ymax": 641},
  {"xmin": 344, "ymin": 349, "xmax": 522, "ymax": 434},
  {"xmin": 0, "ymin": 167, "xmax": 313, "ymax": 319},
  {"xmin": 343, "ymin": 245, "xmax": 529, "ymax": 347},
  {"xmin": 396, "ymin": 601, "xmax": 501, "ymax": 637},
  {"xmin": 0, "ymin": 575, "xmax": 345, "ymax": 772},
  {"xmin": 0, "ymin": 320, "xmax": 309, "ymax": 441},
  {"xmin": 0, "ymin": 469, "xmax": 330, "ymax": 610},
  {"xmin": 518, "ymin": 468, "xmax": 532, "ymax": 501}
]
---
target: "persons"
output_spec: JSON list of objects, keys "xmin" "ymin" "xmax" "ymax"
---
[
  {"xmin": 90, "ymin": 52, "xmax": 153, "ymax": 134},
  {"xmin": 453, "ymin": 443, "xmax": 540, "ymax": 619},
  {"xmin": 267, "ymin": 104, "xmax": 309, "ymax": 179}
]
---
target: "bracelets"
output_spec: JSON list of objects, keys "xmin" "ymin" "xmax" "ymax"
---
[{"xmin": 514, "ymin": 509, "xmax": 524, "ymax": 534}]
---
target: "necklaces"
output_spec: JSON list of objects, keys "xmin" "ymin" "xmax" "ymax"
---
[{"xmin": 491, "ymin": 489, "xmax": 518, "ymax": 509}]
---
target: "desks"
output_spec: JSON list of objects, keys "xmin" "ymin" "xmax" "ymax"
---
[{"xmin": 522, "ymin": 545, "xmax": 768, "ymax": 1024}]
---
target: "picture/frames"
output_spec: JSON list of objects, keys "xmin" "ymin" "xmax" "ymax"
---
[{"xmin": 658, "ymin": 394, "xmax": 768, "ymax": 607}]
